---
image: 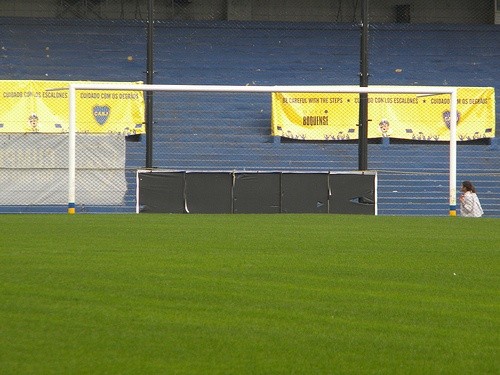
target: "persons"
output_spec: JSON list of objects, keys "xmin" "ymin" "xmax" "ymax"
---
[{"xmin": 460, "ymin": 181, "xmax": 484, "ymax": 217}]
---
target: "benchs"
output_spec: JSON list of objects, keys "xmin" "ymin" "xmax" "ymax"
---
[{"xmin": 1, "ymin": 16, "xmax": 499, "ymax": 218}]
